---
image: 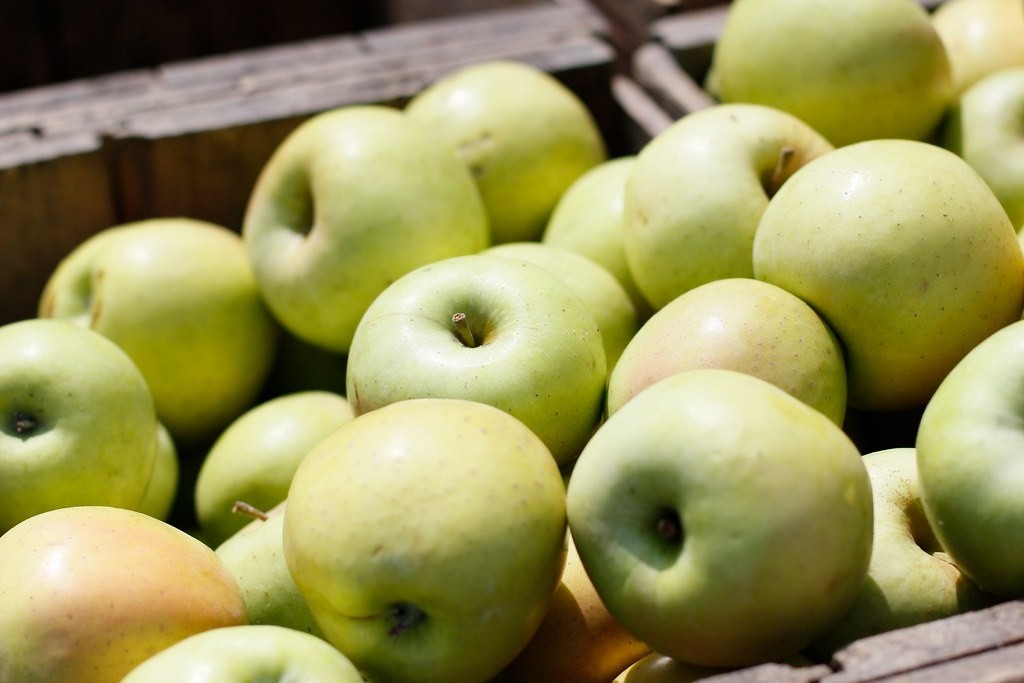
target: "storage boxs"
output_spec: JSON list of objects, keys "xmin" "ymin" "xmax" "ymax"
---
[{"xmin": 0, "ymin": 0, "xmax": 1024, "ymax": 683}]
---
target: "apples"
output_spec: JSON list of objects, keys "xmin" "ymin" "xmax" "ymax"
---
[{"xmin": 0, "ymin": 0, "xmax": 1024, "ymax": 683}]
